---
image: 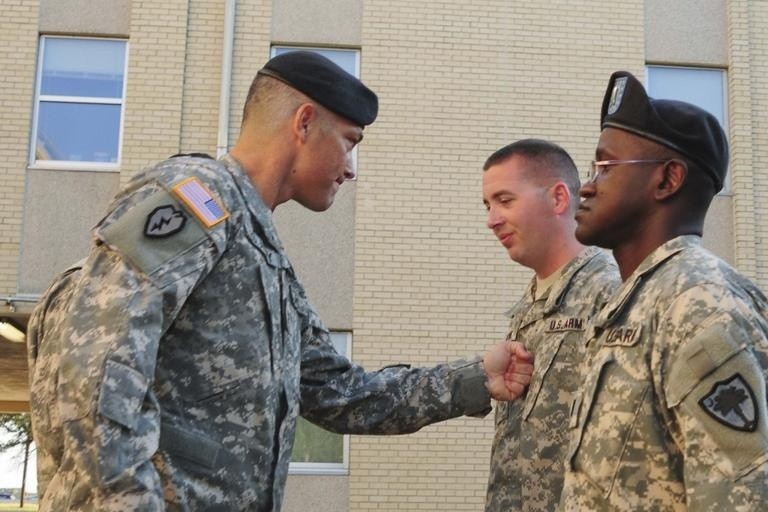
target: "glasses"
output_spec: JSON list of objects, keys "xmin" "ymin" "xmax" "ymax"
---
[{"xmin": 587, "ymin": 159, "xmax": 666, "ymax": 184}]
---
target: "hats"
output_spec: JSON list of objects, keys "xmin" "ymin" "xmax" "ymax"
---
[
  {"xmin": 601, "ymin": 71, "xmax": 729, "ymax": 193},
  {"xmin": 258, "ymin": 51, "xmax": 378, "ymax": 127}
]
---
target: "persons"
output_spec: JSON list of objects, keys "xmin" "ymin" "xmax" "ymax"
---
[
  {"xmin": 28, "ymin": 151, "xmax": 219, "ymax": 510},
  {"xmin": 558, "ymin": 68, "xmax": 768, "ymax": 510},
  {"xmin": 27, "ymin": 49, "xmax": 536, "ymax": 511},
  {"xmin": 477, "ymin": 140, "xmax": 623, "ymax": 511}
]
